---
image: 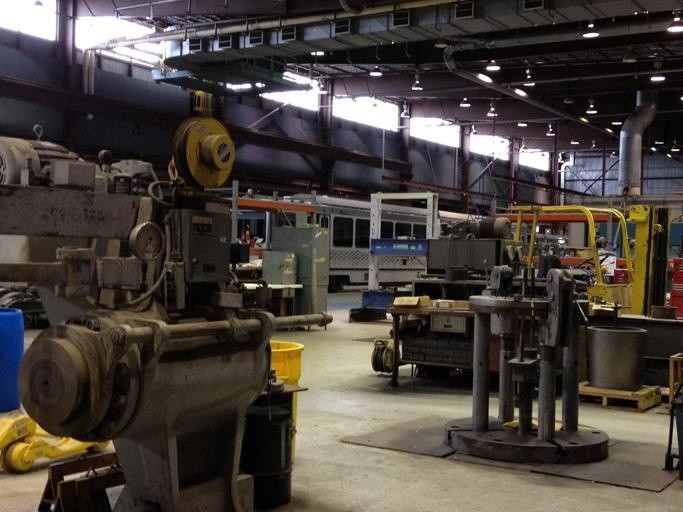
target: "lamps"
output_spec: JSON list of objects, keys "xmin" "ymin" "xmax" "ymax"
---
[
  {"xmin": 411, "ymin": 79, "xmax": 422, "ymax": 90},
  {"xmin": 582, "ymin": 24, "xmax": 600, "ymax": 38},
  {"xmin": 667, "ymin": 17, "xmax": 682, "ymax": 32},
  {"xmin": 486, "ymin": 60, "xmax": 500, "ymax": 72},
  {"xmin": 371, "ymin": 65, "xmax": 383, "ymax": 78},
  {"xmin": 460, "ymin": 99, "xmax": 471, "ymax": 107},
  {"xmin": 522, "ymin": 75, "xmax": 535, "ymax": 86}
]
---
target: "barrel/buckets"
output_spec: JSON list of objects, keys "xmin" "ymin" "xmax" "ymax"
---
[
  {"xmin": 613, "ymin": 269, "xmax": 633, "ymax": 284},
  {"xmin": 671, "ymin": 258, "xmax": 683, "ymax": 317},
  {"xmin": 0, "ymin": 308, "xmax": 25, "ymax": 414}
]
